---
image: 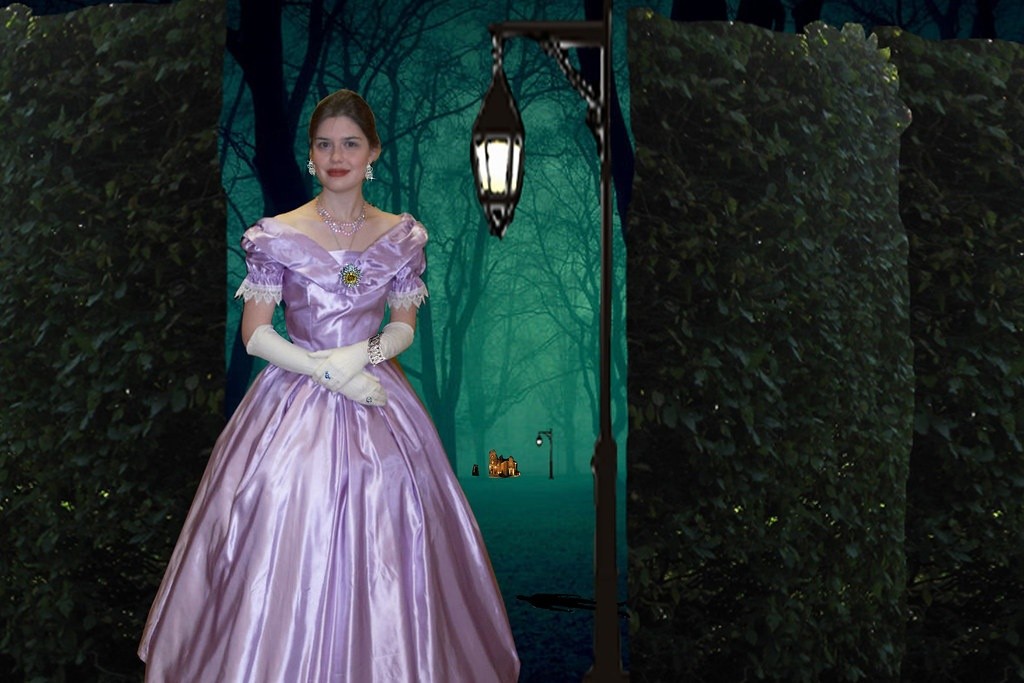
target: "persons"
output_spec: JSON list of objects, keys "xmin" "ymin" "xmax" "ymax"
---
[{"xmin": 137, "ymin": 89, "xmax": 518, "ymax": 683}]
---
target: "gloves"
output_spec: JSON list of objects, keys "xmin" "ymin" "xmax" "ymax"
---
[
  {"xmin": 308, "ymin": 322, "xmax": 414, "ymax": 392},
  {"xmin": 246, "ymin": 325, "xmax": 387, "ymax": 407}
]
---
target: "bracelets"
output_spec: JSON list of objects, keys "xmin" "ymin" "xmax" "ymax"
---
[{"xmin": 368, "ymin": 330, "xmax": 384, "ymax": 365}]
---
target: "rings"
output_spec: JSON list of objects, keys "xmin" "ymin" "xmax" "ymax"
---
[
  {"xmin": 325, "ymin": 372, "xmax": 331, "ymax": 380},
  {"xmin": 366, "ymin": 396, "xmax": 372, "ymax": 404}
]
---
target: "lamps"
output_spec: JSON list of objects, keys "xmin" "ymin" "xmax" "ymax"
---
[
  {"xmin": 536, "ymin": 435, "xmax": 543, "ymax": 447},
  {"xmin": 469, "ymin": 38, "xmax": 526, "ymax": 240}
]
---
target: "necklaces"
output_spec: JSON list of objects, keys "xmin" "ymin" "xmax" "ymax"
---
[
  {"xmin": 314, "ymin": 196, "xmax": 367, "ymax": 237},
  {"xmin": 319, "ymin": 198, "xmax": 364, "ymax": 249}
]
